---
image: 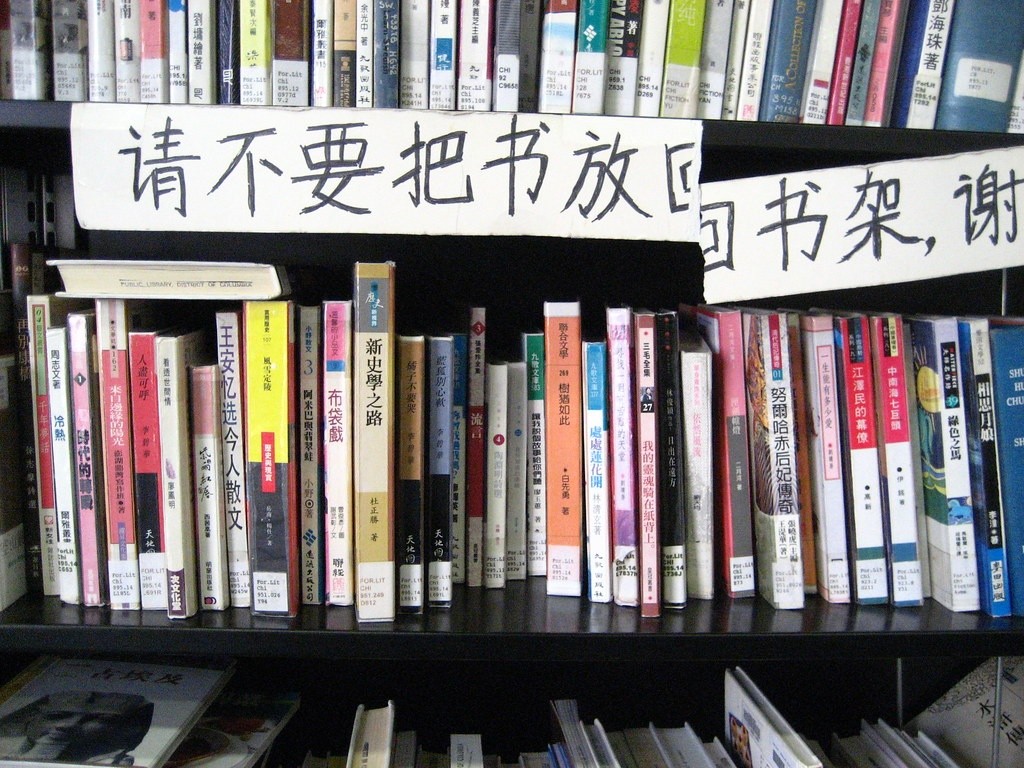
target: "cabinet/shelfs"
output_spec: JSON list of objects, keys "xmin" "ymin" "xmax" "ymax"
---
[{"xmin": 0, "ymin": 101, "xmax": 1024, "ymax": 768}]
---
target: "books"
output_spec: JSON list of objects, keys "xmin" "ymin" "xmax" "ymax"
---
[
  {"xmin": 0, "ymin": 256, "xmax": 1024, "ymax": 768},
  {"xmin": 0, "ymin": 0, "xmax": 1024, "ymax": 138}
]
